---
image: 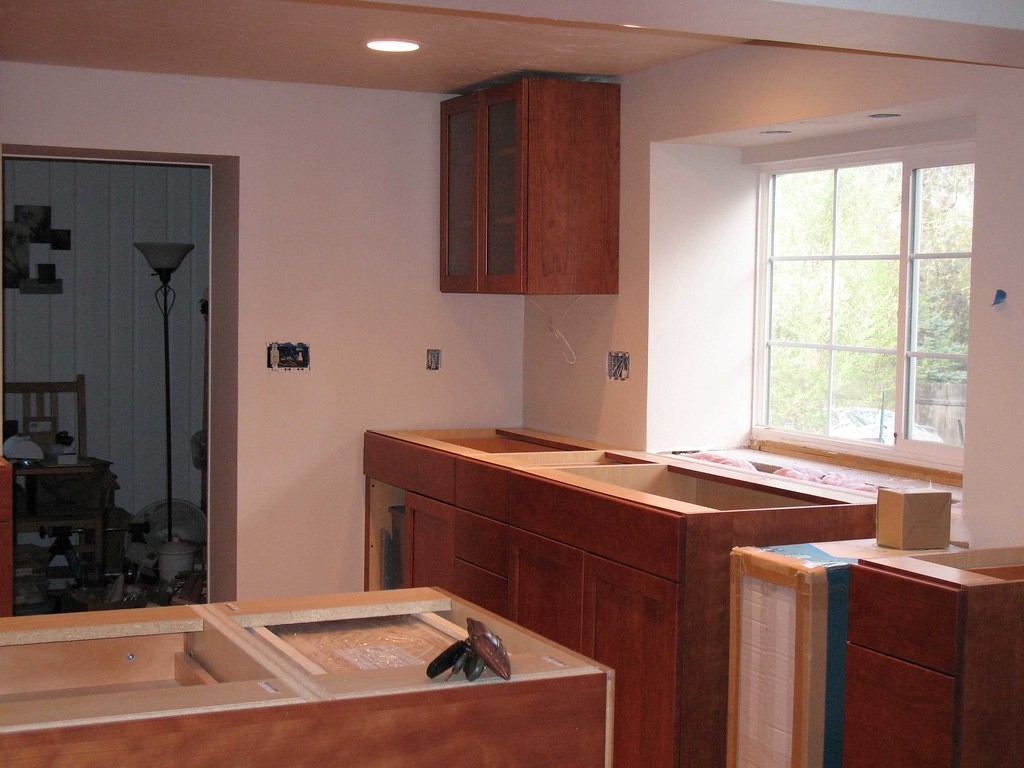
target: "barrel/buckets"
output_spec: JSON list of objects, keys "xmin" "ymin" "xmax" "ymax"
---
[{"xmin": 158, "ymin": 553, "xmax": 193, "ymax": 583}]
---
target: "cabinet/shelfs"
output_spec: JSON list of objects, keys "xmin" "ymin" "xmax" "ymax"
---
[
  {"xmin": 451, "ymin": 507, "xmax": 510, "ymax": 622},
  {"xmin": 506, "ymin": 507, "xmax": 876, "ymax": 767},
  {"xmin": 843, "ymin": 545, "xmax": 1024, "ymax": 768},
  {"xmin": 438, "ymin": 76, "xmax": 618, "ymax": 294},
  {"xmin": 364, "ymin": 475, "xmax": 454, "ymax": 595}
]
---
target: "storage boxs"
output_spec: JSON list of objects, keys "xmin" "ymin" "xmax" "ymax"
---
[
  {"xmin": 724, "ymin": 538, "xmax": 968, "ymax": 767},
  {"xmin": 876, "ymin": 485, "xmax": 953, "ymax": 550}
]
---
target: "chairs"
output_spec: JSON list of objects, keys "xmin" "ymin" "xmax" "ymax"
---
[{"xmin": 5, "ymin": 374, "xmax": 104, "ymax": 583}]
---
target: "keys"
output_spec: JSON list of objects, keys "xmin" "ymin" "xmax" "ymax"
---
[{"xmin": 425, "ymin": 639, "xmax": 486, "ymax": 681}]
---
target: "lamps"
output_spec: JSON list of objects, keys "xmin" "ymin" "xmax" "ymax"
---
[{"xmin": 127, "ymin": 241, "xmax": 195, "ymax": 542}]
